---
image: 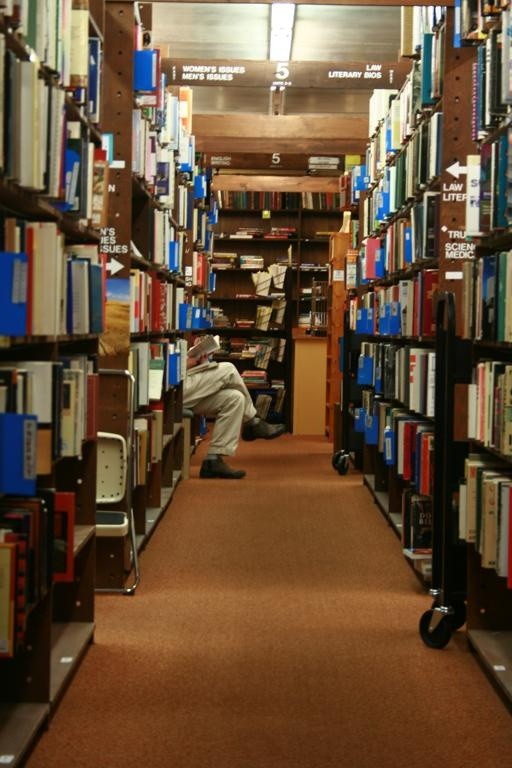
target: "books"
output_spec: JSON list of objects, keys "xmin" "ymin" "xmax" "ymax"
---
[
  {"xmin": 214, "ymin": 191, "xmax": 342, "ymax": 420},
  {"xmin": 1, "ymin": 0, "xmax": 220, "ymax": 662},
  {"xmin": 339, "ymin": 0, "xmax": 512, "ymax": 590}
]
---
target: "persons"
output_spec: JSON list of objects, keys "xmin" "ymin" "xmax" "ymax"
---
[{"xmin": 184, "ymin": 361, "xmax": 285, "ymax": 479}]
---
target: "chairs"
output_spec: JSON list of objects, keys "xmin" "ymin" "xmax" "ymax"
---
[{"xmin": 96, "ymin": 368, "xmax": 140, "ymax": 596}]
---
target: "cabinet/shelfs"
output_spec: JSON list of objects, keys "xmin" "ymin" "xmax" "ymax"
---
[
  {"xmin": 212, "ymin": 200, "xmax": 358, "ymax": 434},
  {"xmin": 1, "ymin": 0, "xmax": 99, "ymax": 767},
  {"xmin": 341, "ymin": 0, "xmax": 511, "ymax": 711},
  {"xmin": 326, "ymin": 231, "xmax": 359, "ymax": 443},
  {"xmin": 94, "ymin": 1, "xmax": 213, "ymax": 589}
]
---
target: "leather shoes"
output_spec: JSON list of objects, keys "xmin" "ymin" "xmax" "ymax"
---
[
  {"xmin": 242, "ymin": 417, "xmax": 286, "ymax": 441},
  {"xmin": 201, "ymin": 458, "xmax": 245, "ymax": 478}
]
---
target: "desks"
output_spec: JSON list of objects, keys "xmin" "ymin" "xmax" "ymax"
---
[{"xmin": 291, "ymin": 327, "xmax": 328, "ymax": 436}]
